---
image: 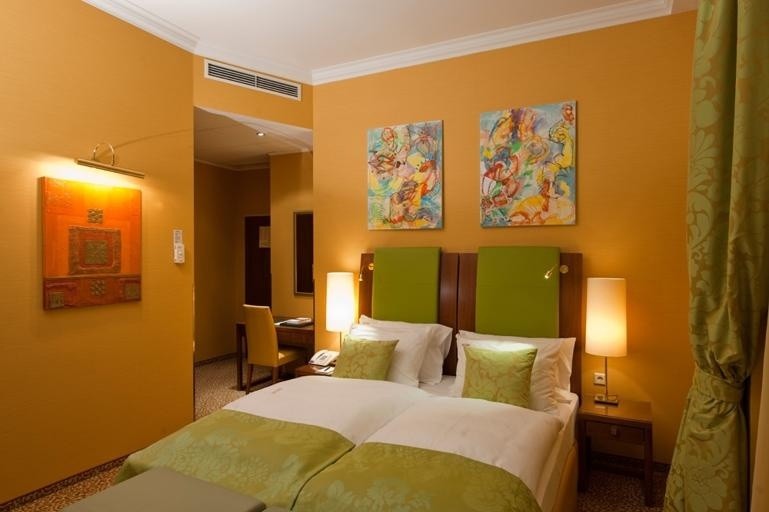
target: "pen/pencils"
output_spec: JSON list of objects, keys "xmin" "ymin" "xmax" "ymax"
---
[{"xmin": 324, "ymin": 367, "xmax": 330, "ymax": 371}]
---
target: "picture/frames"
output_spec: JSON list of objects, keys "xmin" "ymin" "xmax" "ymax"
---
[{"xmin": 293, "ymin": 211, "xmax": 313, "ymax": 296}]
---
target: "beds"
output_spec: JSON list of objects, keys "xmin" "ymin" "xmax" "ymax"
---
[{"xmin": 126, "ymin": 245, "xmax": 584, "ymax": 512}]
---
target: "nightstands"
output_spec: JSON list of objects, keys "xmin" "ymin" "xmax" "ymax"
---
[
  {"xmin": 294, "ymin": 360, "xmax": 337, "ymax": 378},
  {"xmin": 576, "ymin": 395, "xmax": 653, "ymax": 506}
]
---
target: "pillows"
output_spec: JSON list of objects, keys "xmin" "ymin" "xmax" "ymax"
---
[{"xmin": 332, "ymin": 313, "xmax": 577, "ymax": 417}]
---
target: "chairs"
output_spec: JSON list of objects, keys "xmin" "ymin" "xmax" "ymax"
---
[{"xmin": 242, "ymin": 303, "xmax": 304, "ymax": 395}]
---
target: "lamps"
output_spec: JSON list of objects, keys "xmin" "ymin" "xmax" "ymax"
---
[
  {"xmin": 584, "ymin": 277, "xmax": 628, "ymax": 405},
  {"xmin": 325, "ymin": 271, "xmax": 356, "ymax": 354},
  {"xmin": 76, "ymin": 142, "xmax": 145, "ymax": 180}
]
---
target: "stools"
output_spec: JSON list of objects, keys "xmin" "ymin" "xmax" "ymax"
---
[{"xmin": 58, "ymin": 465, "xmax": 290, "ymax": 511}]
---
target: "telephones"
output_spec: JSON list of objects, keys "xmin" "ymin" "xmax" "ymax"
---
[{"xmin": 308, "ymin": 350, "xmax": 340, "ymax": 366}]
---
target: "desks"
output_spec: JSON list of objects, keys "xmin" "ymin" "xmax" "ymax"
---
[{"xmin": 235, "ymin": 317, "xmax": 314, "ymax": 391}]
